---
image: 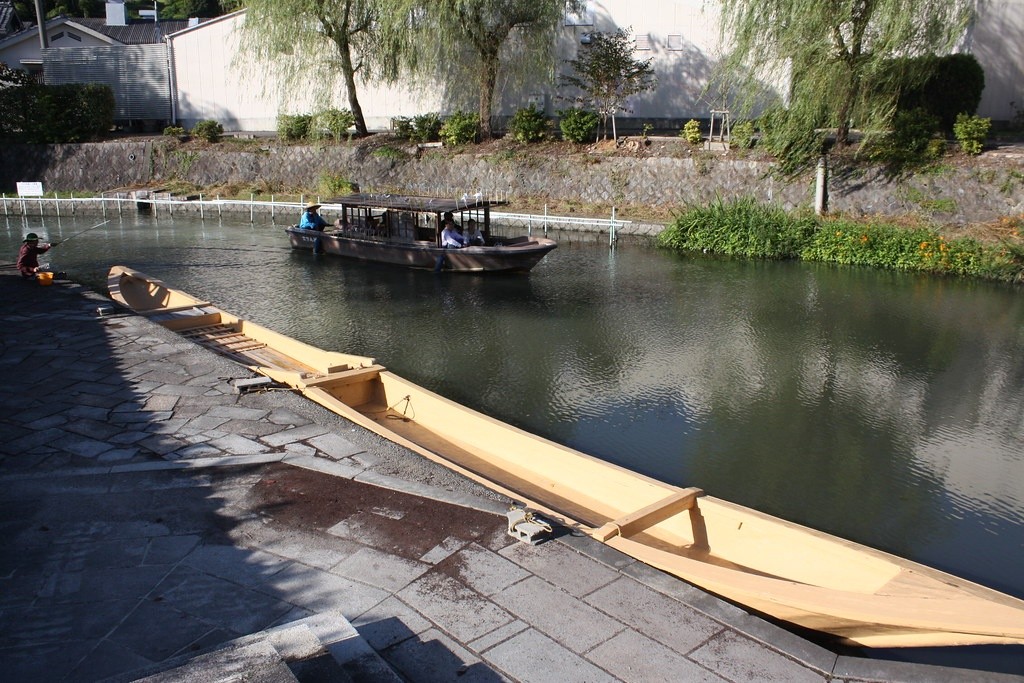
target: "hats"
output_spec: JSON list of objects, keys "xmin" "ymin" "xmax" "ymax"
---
[
  {"xmin": 23, "ymin": 233, "xmax": 43, "ymax": 242},
  {"xmin": 305, "ymin": 201, "xmax": 322, "ymax": 209}
]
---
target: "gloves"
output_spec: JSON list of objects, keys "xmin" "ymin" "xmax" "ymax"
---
[{"xmin": 50, "ymin": 242, "xmax": 57, "ymax": 246}]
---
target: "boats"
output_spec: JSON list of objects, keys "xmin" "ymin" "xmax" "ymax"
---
[
  {"xmin": 108, "ymin": 264, "xmax": 1024, "ymax": 648},
  {"xmin": 283, "ymin": 192, "xmax": 559, "ymax": 275}
]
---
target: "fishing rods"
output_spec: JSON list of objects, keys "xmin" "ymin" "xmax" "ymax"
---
[{"xmin": 51, "ymin": 214, "xmax": 120, "ymax": 248}]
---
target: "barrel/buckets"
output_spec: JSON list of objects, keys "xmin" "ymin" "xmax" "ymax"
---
[{"xmin": 35, "ymin": 272, "xmax": 54, "ymax": 286}]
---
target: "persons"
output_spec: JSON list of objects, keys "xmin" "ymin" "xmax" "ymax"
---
[
  {"xmin": 16, "ymin": 233, "xmax": 59, "ymax": 281},
  {"xmin": 300, "ymin": 201, "xmax": 330, "ymax": 232},
  {"xmin": 439, "ymin": 210, "xmax": 485, "ymax": 248},
  {"xmin": 365, "ymin": 210, "xmax": 415, "ymax": 240}
]
